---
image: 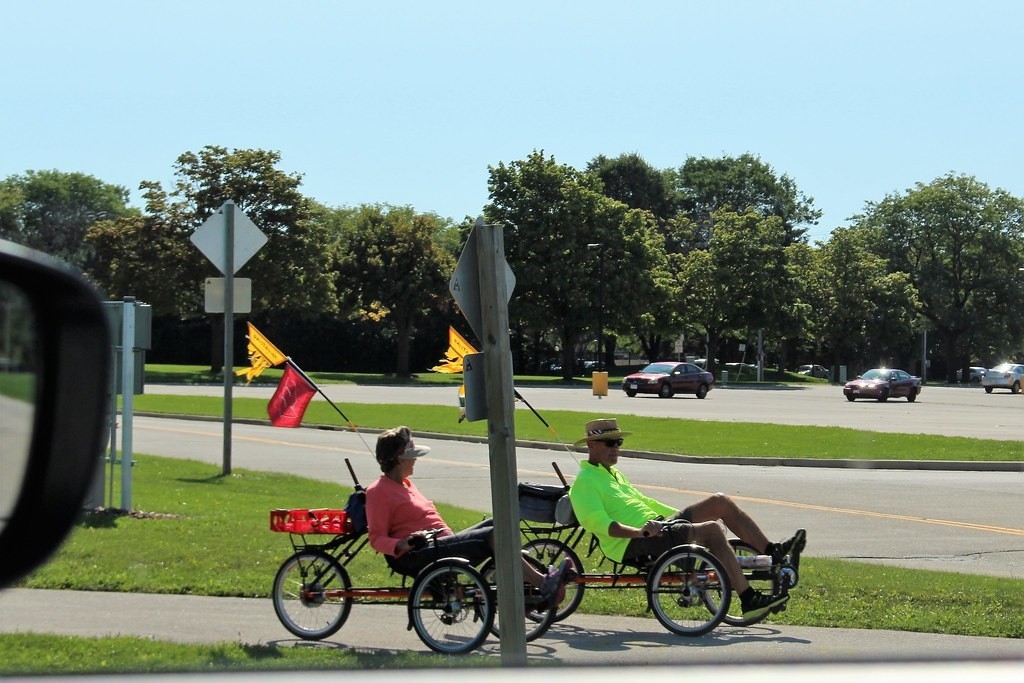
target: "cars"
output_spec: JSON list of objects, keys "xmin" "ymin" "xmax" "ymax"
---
[
  {"xmin": 622, "ymin": 361, "xmax": 714, "ymax": 401},
  {"xmin": 843, "ymin": 369, "xmax": 921, "ymax": 402},
  {"xmin": 956, "ymin": 364, "xmax": 1024, "ymax": 394},
  {"xmin": 798, "ymin": 364, "xmax": 830, "ymax": 377}
]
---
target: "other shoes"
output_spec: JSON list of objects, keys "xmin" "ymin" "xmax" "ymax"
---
[{"xmin": 767, "ymin": 529, "xmax": 806, "ymax": 570}]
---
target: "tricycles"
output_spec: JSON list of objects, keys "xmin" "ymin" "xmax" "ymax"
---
[{"xmin": 270, "ymin": 457, "xmax": 807, "ymax": 655}]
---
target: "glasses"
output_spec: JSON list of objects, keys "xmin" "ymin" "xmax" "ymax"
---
[{"xmin": 594, "ymin": 439, "xmax": 624, "ymax": 447}]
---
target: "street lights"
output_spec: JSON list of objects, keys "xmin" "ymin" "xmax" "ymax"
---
[{"xmin": 587, "ymin": 243, "xmax": 604, "ymax": 372}]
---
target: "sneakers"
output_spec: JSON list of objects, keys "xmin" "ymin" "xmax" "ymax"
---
[
  {"xmin": 740, "ymin": 587, "xmax": 790, "ymax": 624},
  {"xmin": 533, "ymin": 584, "xmax": 565, "ymax": 612},
  {"xmin": 540, "ymin": 557, "xmax": 573, "ymax": 599}
]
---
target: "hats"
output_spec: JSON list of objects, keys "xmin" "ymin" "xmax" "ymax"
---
[
  {"xmin": 377, "ymin": 425, "xmax": 431, "ymax": 463},
  {"xmin": 573, "ymin": 418, "xmax": 632, "ymax": 449}
]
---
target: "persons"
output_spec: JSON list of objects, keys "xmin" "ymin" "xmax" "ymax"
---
[
  {"xmin": 570, "ymin": 419, "xmax": 806, "ymax": 623},
  {"xmin": 365, "ymin": 426, "xmax": 573, "ymax": 614}
]
